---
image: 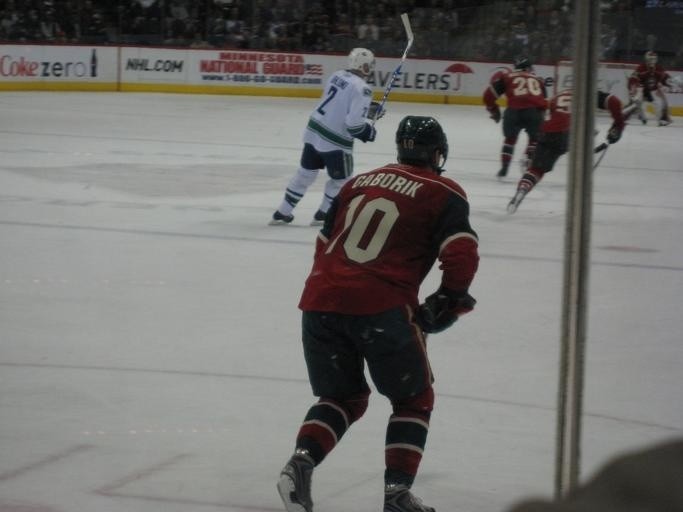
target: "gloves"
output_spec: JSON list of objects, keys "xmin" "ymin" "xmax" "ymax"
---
[
  {"xmin": 492, "ymin": 110, "xmax": 501, "ymax": 123},
  {"xmin": 367, "ymin": 101, "xmax": 387, "ymax": 120},
  {"xmin": 415, "ymin": 287, "xmax": 477, "ymax": 333},
  {"xmin": 608, "ymin": 124, "xmax": 622, "ymax": 144},
  {"xmin": 355, "ymin": 122, "xmax": 376, "ymax": 142}
]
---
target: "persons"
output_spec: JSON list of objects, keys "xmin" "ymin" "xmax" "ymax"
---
[
  {"xmin": 271, "ymin": 47, "xmax": 385, "ymax": 223},
  {"xmin": 481, "ymin": 55, "xmax": 548, "ymax": 177},
  {"xmin": 627, "ymin": 50, "xmax": 672, "ymax": 126},
  {"xmin": 277, "ymin": 114, "xmax": 479, "ymax": 512},
  {"xmin": 2, "ymin": 0, "xmax": 682, "ymax": 65},
  {"xmin": 505, "ymin": 89, "xmax": 625, "ymax": 212}
]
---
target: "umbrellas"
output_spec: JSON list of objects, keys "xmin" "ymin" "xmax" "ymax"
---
[{"xmin": 443, "ymin": 63, "xmax": 474, "ymax": 91}]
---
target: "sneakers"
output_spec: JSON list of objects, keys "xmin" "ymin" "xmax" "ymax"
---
[
  {"xmin": 384, "ymin": 484, "xmax": 436, "ymax": 512},
  {"xmin": 273, "ymin": 211, "xmax": 294, "ymax": 222},
  {"xmin": 314, "ymin": 210, "xmax": 326, "ymax": 221},
  {"xmin": 276, "ymin": 453, "xmax": 315, "ymax": 511}
]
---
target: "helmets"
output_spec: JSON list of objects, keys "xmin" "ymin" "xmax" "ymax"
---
[
  {"xmin": 395, "ymin": 115, "xmax": 446, "ymax": 166},
  {"xmin": 646, "ymin": 51, "xmax": 659, "ymax": 68},
  {"xmin": 514, "ymin": 55, "xmax": 531, "ymax": 69},
  {"xmin": 345, "ymin": 48, "xmax": 374, "ymax": 76}
]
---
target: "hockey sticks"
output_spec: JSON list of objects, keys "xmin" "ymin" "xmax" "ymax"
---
[{"xmin": 371, "ymin": 13, "xmax": 414, "ymax": 127}]
